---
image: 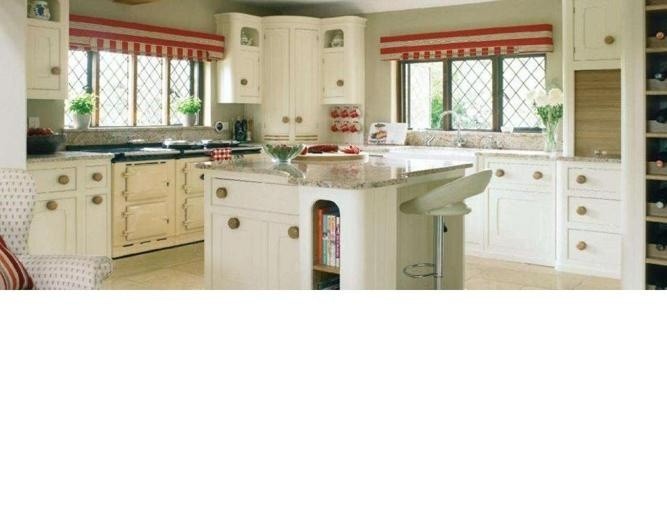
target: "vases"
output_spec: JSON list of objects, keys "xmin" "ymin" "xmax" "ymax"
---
[{"xmin": 541, "ymin": 120, "xmax": 560, "ymax": 153}]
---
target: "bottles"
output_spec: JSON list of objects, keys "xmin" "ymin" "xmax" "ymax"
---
[
  {"xmin": 653, "ymin": 28, "xmax": 667, "ymax": 291},
  {"xmin": 232, "ymin": 115, "xmax": 244, "ymax": 141}
]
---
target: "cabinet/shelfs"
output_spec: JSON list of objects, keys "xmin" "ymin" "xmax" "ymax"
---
[
  {"xmin": 408, "ymin": 159, "xmax": 557, "ymax": 267},
  {"xmin": 26, "ymin": 0, "xmax": 68, "ymax": 100},
  {"xmin": 562, "ymin": 1, "xmax": 622, "ymax": 71},
  {"xmin": 27, "ymin": 158, "xmax": 112, "ymax": 258},
  {"xmin": 556, "ymin": 162, "xmax": 623, "ymax": 280},
  {"xmin": 647, "ymin": 4, "xmax": 667, "ymax": 266},
  {"xmin": 112, "ymin": 154, "xmax": 260, "ymax": 259},
  {"xmin": 213, "ymin": 12, "xmax": 368, "ymax": 147},
  {"xmin": 202, "ymin": 170, "xmax": 397, "ymax": 290}
]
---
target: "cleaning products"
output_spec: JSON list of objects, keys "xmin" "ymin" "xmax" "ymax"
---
[
  {"xmin": 241, "ymin": 114, "xmax": 247, "ymax": 141},
  {"xmin": 234, "ymin": 113, "xmax": 242, "ymax": 140},
  {"xmin": 246, "ymin": 113, "xmax": 253, "ymax": 141},
  {"xmin": 230, "ymin": 117, "xmax": 235, "ymax": 140}
]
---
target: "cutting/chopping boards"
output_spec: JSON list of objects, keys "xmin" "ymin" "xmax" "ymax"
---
[{"xmin": 297, "ymin": 151, "xmax": 365, "ymax": 159}]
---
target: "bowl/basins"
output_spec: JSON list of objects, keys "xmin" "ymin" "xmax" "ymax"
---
[
  {"xmin": 27, "ymin": 133, "xmax": 66, "ymax": 154},
  {"xmin": 261, "ymin": 143, "xmax": 307, "ymax": 163}
]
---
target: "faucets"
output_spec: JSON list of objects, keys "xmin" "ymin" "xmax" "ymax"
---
[
  {"xmin": 454, "ymin": 125, "xmax": 465, "ymax": 147},
  {"xmin": 488, "ymin": 135, "xmax": 502, "ymax": 149},
  {"xmin": 426, "ymin": 136, "xmax": 435, "ymax": 145}
]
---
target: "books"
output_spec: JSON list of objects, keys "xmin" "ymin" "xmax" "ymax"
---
[{"xmin": 315, "ymin": 205, "xmax": 342, "ymax": 267}]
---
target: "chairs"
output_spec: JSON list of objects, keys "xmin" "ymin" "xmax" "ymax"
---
[{"xmin": 400, "ymin": 170, "xmax": 491, "ymax": 289}]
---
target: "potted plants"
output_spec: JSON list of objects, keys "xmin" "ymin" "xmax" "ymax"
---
[
  {"xmin": 66, "ymin": 93, "xmax": 100, "ymax": 128},
  {"xmin": 174, "ymin": 95, "xmax": 202, "ymax": 127}
]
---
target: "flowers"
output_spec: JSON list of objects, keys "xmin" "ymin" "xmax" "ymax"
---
[{"xmin": 525, "ymin": 87, "xmax": 563, "ymax": 147}]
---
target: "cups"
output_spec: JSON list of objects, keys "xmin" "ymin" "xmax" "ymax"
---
[
  {"xmin": 330, "ymin": 106, "xmax": 362, "ymax": 133},
  {"xmin": 27, "ymin": 0, "xmax": 50, "ymax": 20}
]
